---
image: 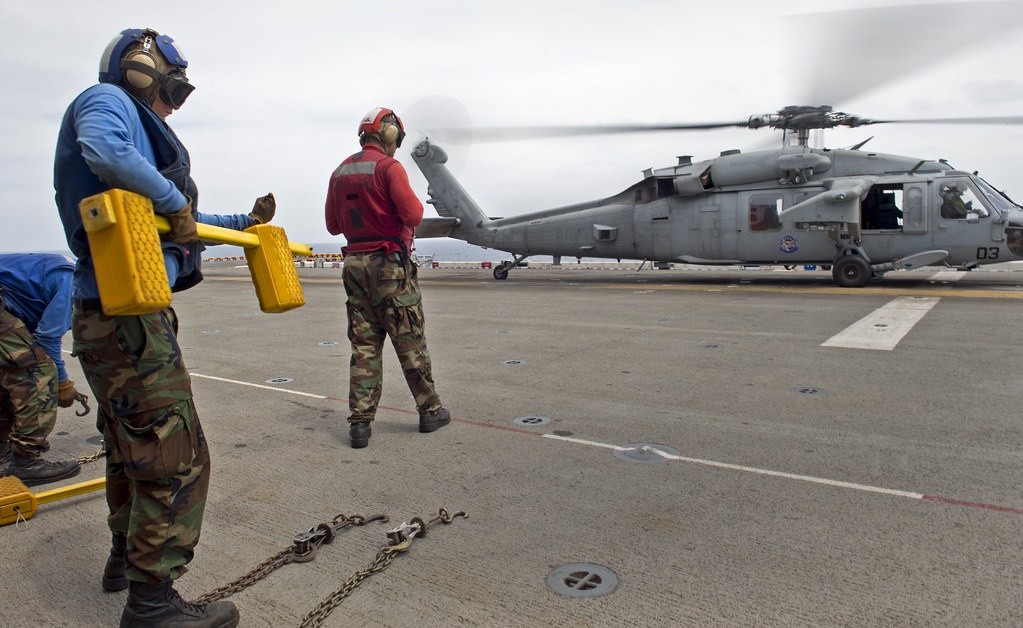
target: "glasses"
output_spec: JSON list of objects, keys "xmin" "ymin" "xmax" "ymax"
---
[
  {"xmin": 395, "ymin": 123, "xmax": 406, "ymax": 148},
  {"xmin": 159, "ymin": 75, "xmax": 196, "ymax": 110}
]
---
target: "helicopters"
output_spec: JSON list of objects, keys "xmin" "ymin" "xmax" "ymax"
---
[{"xmin": 410, "ymin": 97, "xmax": 1022, "ymax": 286}]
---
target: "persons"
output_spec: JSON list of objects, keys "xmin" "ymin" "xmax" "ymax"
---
[
  {"xmin": 0, "ymin": 252, "xmax": 80, "ymax": 486},
  {"xmin": 325, "ymin": 107, "xmax": 446, "ymax": 449},
  {"xmin": 944, "ymin": 183, "xmax": 970, "ymax": 218},
  {"xmin": 52, "ymin": 26, "xmax": 277, "ymax": 628}
]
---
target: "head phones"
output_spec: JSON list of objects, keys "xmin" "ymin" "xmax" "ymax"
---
[
  {"xmin": 359, "ymin": 112, "xmax": 398, "ymax": 147},
  {"xmin": 121, "ymin": 27, "xmax": 161, "ymax": 93}
]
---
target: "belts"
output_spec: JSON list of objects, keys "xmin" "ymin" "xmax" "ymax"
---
[{"xmin": 74, "ymin": 298, "xmax": 101, "ymax": 310}]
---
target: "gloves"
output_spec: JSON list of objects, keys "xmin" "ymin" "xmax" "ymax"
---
[
  {"xmin": 57, "ymin": 380, "xmax": 77, "ymax": 407},
  {"xmin": 248, "ymin": 193, "xmax": 276, "ymax": 224},
  {"xmin": 157, "ymin": 194, "xmax": 197, "ymax": 244}
]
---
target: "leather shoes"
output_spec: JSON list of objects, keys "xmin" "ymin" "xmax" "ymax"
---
[
  {"xmin": 102, "ymin": 548, "xmax": 129, "ymax": 590},
  {"xmin": 6, "ymin": 449, "xmax": 81, "ymax": 487},
  {"xmin": 349, "ymin": 420, "xmax": 371, "ymax": 447},
  {"xmin": 0, "ymin": 440, "xmax": 50, "ymax": 463},
  {"xmin": 419, "ymin": 408, "xmax": 451, "ymax": 432},
  {"xmin": 120, "ymin": 581, "xmax": 240, "ymax": 628}
]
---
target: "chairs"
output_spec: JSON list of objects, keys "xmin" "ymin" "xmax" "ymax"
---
[{"xmin": 942, "ymin": 191, "xmax": 956, "ymax": 219}]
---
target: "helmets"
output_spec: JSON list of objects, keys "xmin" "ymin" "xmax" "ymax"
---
[
  {"xmin": 357, "ymin": 107, "xmax": 404, "ymax": 155},
  {"xmin": 98, "ymin": 28, "xmax": 188, "ymax": 108}
]
